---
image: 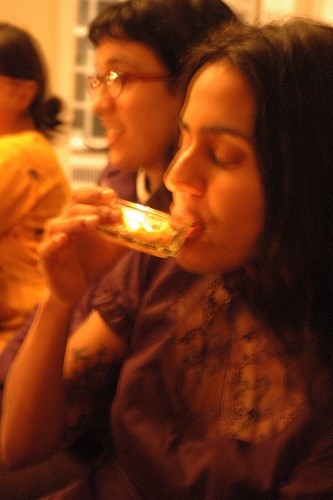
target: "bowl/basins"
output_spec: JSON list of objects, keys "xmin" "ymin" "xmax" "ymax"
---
[{"xmin": 94, "ymin": 200, "xmax": 195, "ymax": 257}]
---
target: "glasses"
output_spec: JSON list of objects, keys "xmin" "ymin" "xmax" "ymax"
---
[{"xmin": 88, "ymin": 69, "xmax": 172, "ymax": 100}]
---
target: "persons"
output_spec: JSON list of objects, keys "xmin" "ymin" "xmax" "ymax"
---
[
  {"xmin": 0, "ymin": 21, "xmax": 69, "ymax": 379},
  {"xmin": 0, "ymin": 18, "xmax": 333, "ymax": 500},
  {"xmin": 61, "ymin": 0, "xmax": 243, "ymax": 401}
]
